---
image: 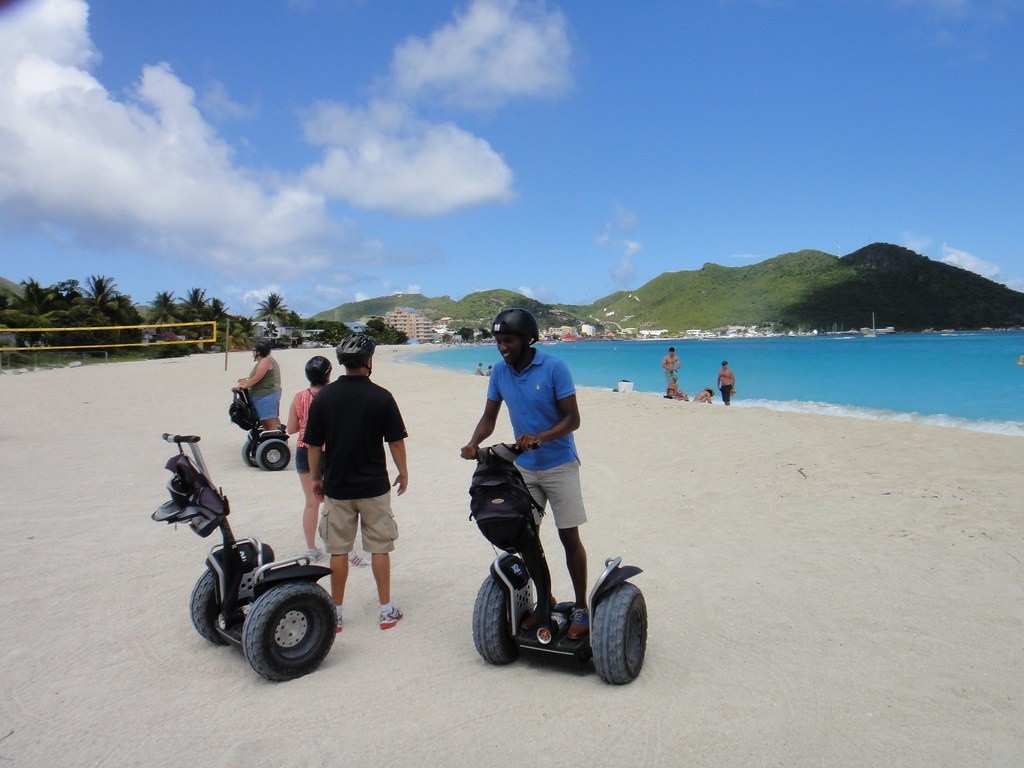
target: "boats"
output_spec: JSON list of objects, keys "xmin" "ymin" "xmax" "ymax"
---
[
  {"xmin": 860, "ymin": 326, "xmax": 896, "ymax": 335},
  {"xmin": 1017, "ymin": 356, "xmax": 1024, "ymax": 366}
]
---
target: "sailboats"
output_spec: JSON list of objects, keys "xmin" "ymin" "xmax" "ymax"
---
[{"xmin": 788, "ymin": 311, "xmax": 876, "ymax": 338}]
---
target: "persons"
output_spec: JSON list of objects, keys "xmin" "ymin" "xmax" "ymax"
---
[
  {"xmin": 236, "ymin": 341, "xmax": 283, "ymax": 431},
  {"xmin": 475, "ymin": 363, "xmax": 493, "ymax": 376},
  {"xmin": 288, "ymin": 356, "xmax": 367, "ymax": 567},
  {"xmin": 663, "ymin": 347, "xmax": 735, "ymax": 406},
  {"xmin": 460, "ymin": 309, "xmax": 590, "ymax": 639},
  {"xmin": 302, "ymin": 333, "xmax": 409, "ymax": 633}
]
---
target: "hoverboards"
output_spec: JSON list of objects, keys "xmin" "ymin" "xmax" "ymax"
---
[
  {"xmin": 231, "ymin": 387, "xmax": 292, "ymax": 471},
  {"xmin": 160, "ymin": 432, "xmax": 339, "ymax": 681},
  {"xmin": 460, "ymin": 440, "xmax": 647, "ymax": 685}
]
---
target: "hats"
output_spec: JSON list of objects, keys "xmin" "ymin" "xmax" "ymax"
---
[
  {"xmin": 705, "ymin": 386, "xmax": 715, "ymax": 397},
  {"xmin": 253, "ymin": 337, "xmax": 274, "ymax": 349}
]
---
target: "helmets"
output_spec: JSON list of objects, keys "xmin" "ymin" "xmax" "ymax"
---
[
  {"xmin": 305, "ymin": 356, "xmax": 333, "ymax": 385},
  {"xmin": 491, "ymin": 308, "xmax": 541, "ymax": 340},
  {"xmin": 335, "ymin": 332, "xmax": 378, "ymax": 366}
]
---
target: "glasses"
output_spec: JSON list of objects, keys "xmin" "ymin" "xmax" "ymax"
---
[{"xmin": 252, "ymin": 348, "xmax": 260, "ymax": 352}]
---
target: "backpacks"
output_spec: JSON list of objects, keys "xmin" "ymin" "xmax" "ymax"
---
[
  {"xmin": 228, "ymin": 388, "xmax": 260, "ymax": 431},
  {"xmin": 467, "ymin": 442, "xmax": 538, "ymax": 554}
]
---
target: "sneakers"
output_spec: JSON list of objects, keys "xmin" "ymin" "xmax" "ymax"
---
[
  {"xmin": 348, "ymin": 550, "xmax": 371, "ymax": 568},
  {"xmin": 306, "ymin": 547, "xmax": 323, "ymax": 564},
  {"xmin": 379, "ymin": 606, "xmax": 403, "ymax": 630},
  {"xmin": 336, "ymin": 614, "xmax": 342, "ymax": 633}
]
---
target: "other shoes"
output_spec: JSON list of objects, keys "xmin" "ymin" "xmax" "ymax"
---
[
  {"xmin": 521, "ymin": 596, "xmax": 557, "ymax": 630},
  {"xmin": 568, "ymin": 602, "xmax": 589, "ymax": 640}
]
